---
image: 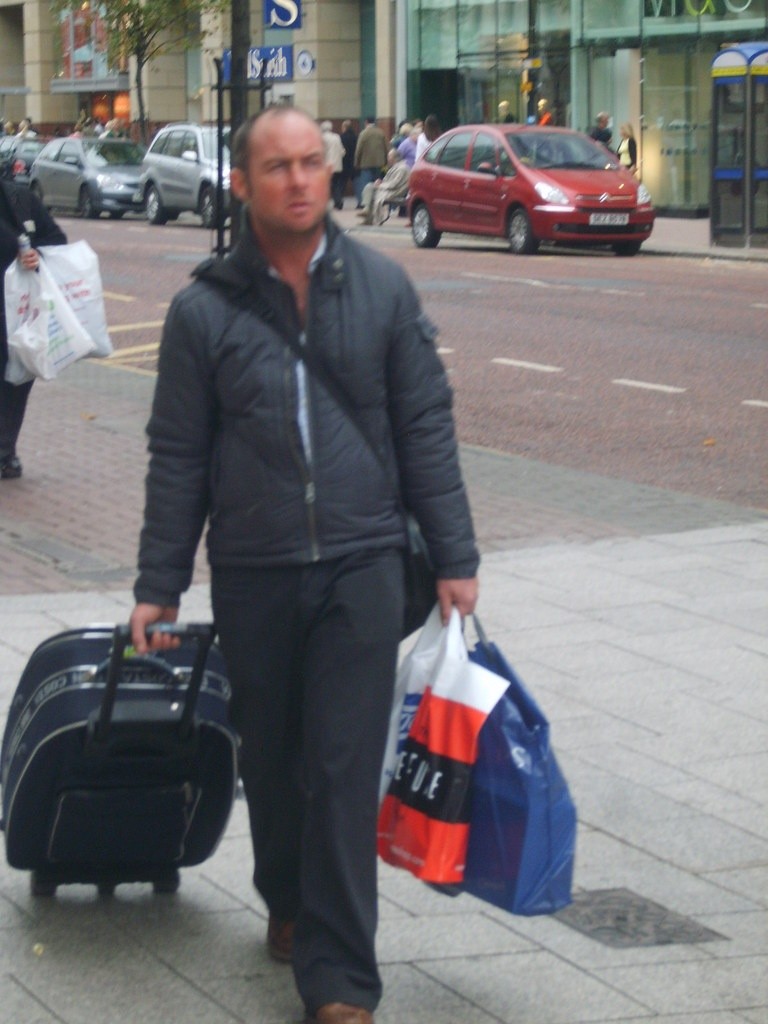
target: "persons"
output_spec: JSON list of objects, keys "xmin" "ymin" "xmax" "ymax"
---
[
  {"xmin": 129, "ymin": 105, "xmax": 481, "ymax": 1024},
  {"xmin": 590, "ymin": 112, "xmax": 613, "ymax": 147},
  {"xmin": 616, "ymin": 123, "xmax": 637, "ymax": 175},
  {"xmin": 538, "ymin": 99, "xmax": 553, "ymax": 127},
  {"xmin": 73, "ymin": 108, "xmax": 130, "ymax": 139},
  {"xmin": 354, "ymin": 116, "xmax": 388, "ymax": 210},
  {"xmin": 499, "ymin": 101, "xmax": 515, "ymax": 122},
  {"xmin": 0, "ymin": 179, "xmax": 67, "ymax": 477},
  {"xmin": 321, "ymin": 119, "xmax": 361, "ymax": 209},
  {"xmin": 5, "ymin": 118, "xmax": 38, "ymax": 138},
  {"xmin": 356, "ymin": 115, "xmax": 442, "ymax": 226}
]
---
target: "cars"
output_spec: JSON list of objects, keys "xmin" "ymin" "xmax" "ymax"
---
[
  {"xmin": 406, "ymin": 122, "xmax": 655, "ymax": 257},
  {"xmin": 0, "ymin": 136, "xmax": 52, "ymax": 189},
  {"xmin": 27, "ymin": 136, "xmax": 151, "ymax": 220}
]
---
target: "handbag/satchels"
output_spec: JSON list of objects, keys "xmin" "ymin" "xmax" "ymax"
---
[
  {"xmin": 373, "ymin": 603, "xmax": 577, "ymax": 916},
  {"xmin": 3, "ymin": 239, "xmax": 114, "ymax": 383},
  {"xmin": 400, "ymin": 515, "xmax": 437, "ymax": 636}
]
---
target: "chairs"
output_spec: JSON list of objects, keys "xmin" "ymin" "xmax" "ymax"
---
[{"xmin": 378, "ymin": 198, "xmax": 403, "ymax": 228}]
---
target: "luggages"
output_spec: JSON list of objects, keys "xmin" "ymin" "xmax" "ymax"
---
[{"xmin": 0, "ymin": 621, "xmax": 238, "ymax": 897}]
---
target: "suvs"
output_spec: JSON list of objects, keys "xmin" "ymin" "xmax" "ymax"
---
[{"xmin": 138, "ymin": 121, "xmax": 236, "ymax": 230}]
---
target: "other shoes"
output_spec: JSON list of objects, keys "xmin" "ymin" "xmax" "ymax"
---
[
  {"xmin": 356, "ymin": 211, "xmax": 367, "ymax": 217},
  {"xmin": 2, "ymin": 457, "xmax": 23, "ymax": 478},
  {"xmin": 314, "ymin": 1001, "xmax": 373, "ymax": 1024},
  {"xmin": 266, "ymin": 913, "xmax": 294, "ymax": 959},
  {"xmin": 359, "ymin": 220, "xmax": 371, "ymax": 224}
]
